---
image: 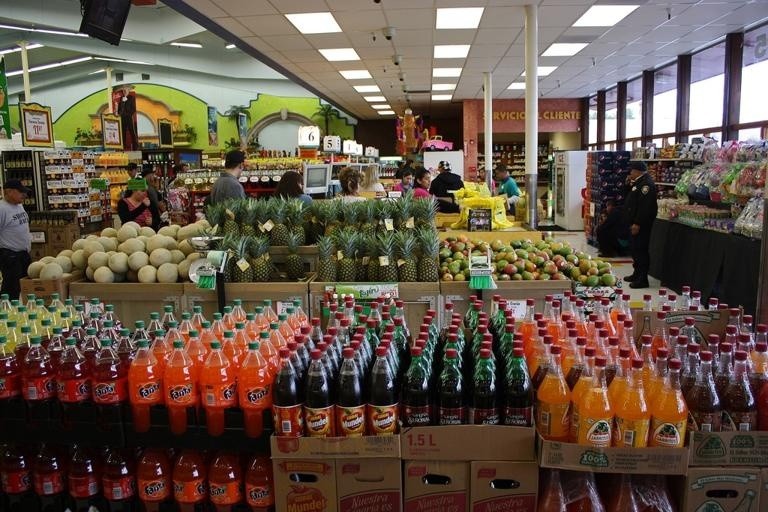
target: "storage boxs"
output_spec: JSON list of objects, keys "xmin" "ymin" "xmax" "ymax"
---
[
  {"xmin": 20, "ymin": 270, "xmax": 84, "ymax": 305},
  {"xmin": 265, "ymin": 459, "xmax": 539, "ymax": 510},
  {"xmin": 680, "ymin": 466, "xmax": 765, "ymax": 511}
]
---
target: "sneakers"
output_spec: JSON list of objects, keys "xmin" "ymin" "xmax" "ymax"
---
[{"xmin": 623, "ymin": 275, "xmax": 648, "ymax": 288}]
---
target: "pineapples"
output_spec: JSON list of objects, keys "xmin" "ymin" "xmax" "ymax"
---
[{"xmin": 206, "ymin": 195, "xmax": 439, "ymax": 282}]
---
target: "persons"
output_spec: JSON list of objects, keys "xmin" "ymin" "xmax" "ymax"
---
[
  {"xmin": 273, "ymin": 170, "xmax": 313, "ymax": 205},
  {"xmin": 411, "ymin": 166, "xmax": 432, "ymax": 200},
  {"xmin": 109, "ymin": 154, "xmax": 191, "ymax": 232},
  {"xmin": 490, "ymin": 164, "xmax": 520, "ymax": 216},
  {"xmin": 209, "ymin": 149, "xmax": 248, "ymax": 205},
  {"xmin": 478, "ymin": 166, "xmax": 496, "ymax": 197},
  {"xmin": 429, "ymin": 160, "xmax": 465, "ymax": 213},
  {"xmin": 622, "ymin": 160, "xmax": 657, "ymax": 288},
  {"xmin": 333, "ymin": 166, "xmax": 363, "ymax": 197},
  {"xmin": 0, "ymin": 179, "xmax": 36, "ymax": 300},
  {"xmin": 394, "ymin": 169, "xmax": 414, "ymax": 197},
  {"xmin": 358, "ymin": 166, "xmax": 388, "ymax": 196}
]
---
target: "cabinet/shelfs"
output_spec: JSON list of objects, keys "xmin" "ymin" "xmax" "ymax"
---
[
  {"xmin": 143, "ymin": 149, "xmax": 205, "ymax": 193},
  {"xmin": 2, "ymin": 149, "xmax": 40, "ymax": 212},
  {"xmin": 94, "ymin": 164, "xmax": 128, "ymax": 214},
  {"xmin": 477, "ymin": 141, "xmax": 549, "ymax": 187},
  {"xmin": 629, "ymin": 158, "xmax": 704, "ymax": 186}
]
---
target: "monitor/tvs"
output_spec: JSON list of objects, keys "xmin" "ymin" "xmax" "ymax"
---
[
  {"xmin": 361, "ymin": 164, "xmax": 378, "ymax": 174},
  {"xmin": 79, "ymin": 0, "xmax": 131, "ymax": 46},
  {"xmin": 303, "ymin": 164, "xmax": 330, "ymax": 194},
  {"xmin": 348, "ymin": 163, "xmax": 361, "ymax": 175},
  {"xmin": 328, "ymin": 162, "xmax": 348, "ymax": 185}
]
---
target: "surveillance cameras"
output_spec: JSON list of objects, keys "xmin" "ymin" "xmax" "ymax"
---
[
  {"xmin": 392, "ymin": 55, "xmax": 401, "ymax": 65},
  {"xmin": 382, "ymin": 27, "xmax": 396, "ymax": 40},
  {"xmin": 397, "ymin": 73, "xmax": 407, "ymax": 81},
  {"xmin": 400, "ymin": 85, "xmax": 410, "ymax": 93},
  {"xmin": 404, "ymin": 95, "xmax": 412, "ymax": 102}
]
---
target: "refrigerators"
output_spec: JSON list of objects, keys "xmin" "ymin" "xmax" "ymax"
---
[{"xmin": 554, "ymin": 151, "xmax": 588, "ymax": 230}]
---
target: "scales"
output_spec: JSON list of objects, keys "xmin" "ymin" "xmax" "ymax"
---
[{"xmin": 187, "ymin": 236, "xmax": 224, "ymax": 283}]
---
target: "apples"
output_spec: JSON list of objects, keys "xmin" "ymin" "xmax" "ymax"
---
[{"xmin": 439, "ymin": 238, "xmax": 615, "ymax": 287}]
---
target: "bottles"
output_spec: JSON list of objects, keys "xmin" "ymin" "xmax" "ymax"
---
[
  {"xmin": 0, "ymin": 287, "xmax": 768, "ymax": 511},
  {"xmin": 381, "ymin": 167, "xmax": 393, "ymax": 176},
  {"xmin": 1, "ymin": 151, "xmax": 169, "ymax": 230},
  {"xmin": 173, "ymin": 158, "xmax": 226, "ymax": 192}
]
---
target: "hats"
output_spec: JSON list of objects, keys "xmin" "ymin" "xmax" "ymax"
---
[
  {"xmin": 438, "ymin": 161, "xmax": 451, "ymax": 170},
  {"xmin": 632, "ymin": 163, "xmax": 646, "ymax": 170},
  {"xmin": 225, "ymin": 151, "xmax": 243, "ymax": 161},
  {"xmin": 4, "ymin": 180, "xmax": 31, "ymax": 191}
]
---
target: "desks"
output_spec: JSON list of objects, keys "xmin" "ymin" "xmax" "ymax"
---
[{"xmin": 647, "ymin": 217, "xmax": 762, "ymax": 327}]
